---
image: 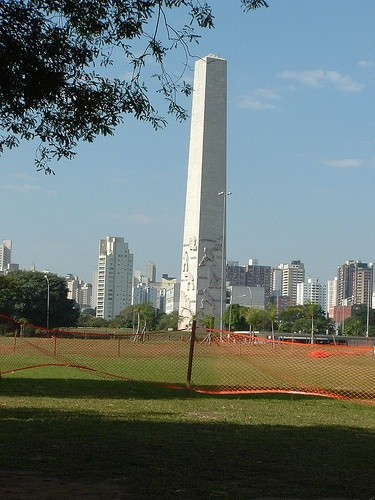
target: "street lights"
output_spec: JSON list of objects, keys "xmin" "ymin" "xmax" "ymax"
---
[
  {"xmin": 217, "ymin": 190, "xmax": 233, "ymax": 341},
  {"xmin": 227, "ymin": 294, "xmax": 247, "ymax": 337},
  {"xmin": 45, "ymin": 275, "xmax": 50, "ymax": 330},
  {"xmin": 342, "ymin": 295, "xmax": 355, "ymax": 336}
]
